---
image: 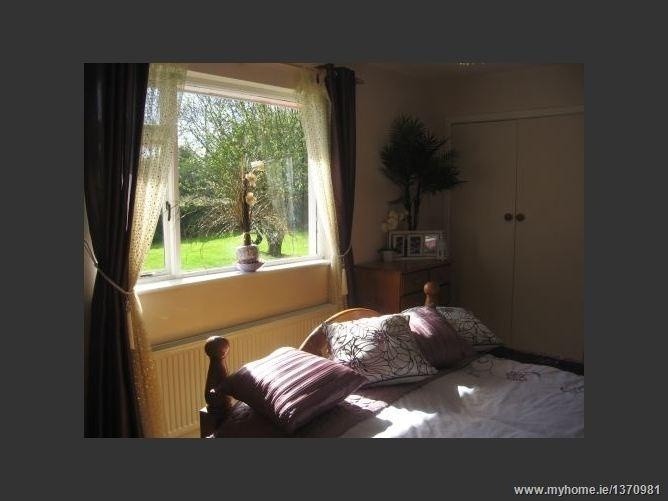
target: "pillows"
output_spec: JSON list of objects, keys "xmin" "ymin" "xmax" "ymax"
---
[
  {"xmin": 223, "ymin": 346, "xmax": 368, "ymax": 436},
  {"xmin": 401, "ymin": 306, "xmax": 504, "ymax": 362},
  {"xmin": 324, "ymin": 315, "xmax": 440, "ymax": 391}
]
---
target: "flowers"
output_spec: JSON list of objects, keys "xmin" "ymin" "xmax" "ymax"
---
[{"xmin": 219, "ymin": 154, "xmax": 270, "ymax": 243}]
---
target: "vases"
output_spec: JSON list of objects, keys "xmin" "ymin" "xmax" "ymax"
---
[
  {"xmin": 378, "ymin": 246, "xmax": 398, "ymax": 264},
  {"xmin": 235, "ymin": 244, "xmax": 264, "ymax": 273}
]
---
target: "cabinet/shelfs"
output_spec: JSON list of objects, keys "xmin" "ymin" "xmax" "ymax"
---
[
  {"xmin": 446, "ymin": 106, "xmax": 582, "ymax": 364},
  {"xmin": 352, "ymin": 259, "xmax": 452, "ymax": 315}
]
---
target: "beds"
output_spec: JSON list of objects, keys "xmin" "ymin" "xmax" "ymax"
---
[{"xmin": 199, "ymin": 281, "xmax": 583, "ymax": 436}]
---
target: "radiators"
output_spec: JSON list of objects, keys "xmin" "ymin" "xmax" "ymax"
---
[{"xmin": 150, "ymin": 303, "xmax": 348, "ymax": 438}]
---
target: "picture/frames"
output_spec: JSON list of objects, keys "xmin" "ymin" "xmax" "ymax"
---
[{"xmin": 389, "ymin": 231, "xmax": 445, "ymax": 258}]
